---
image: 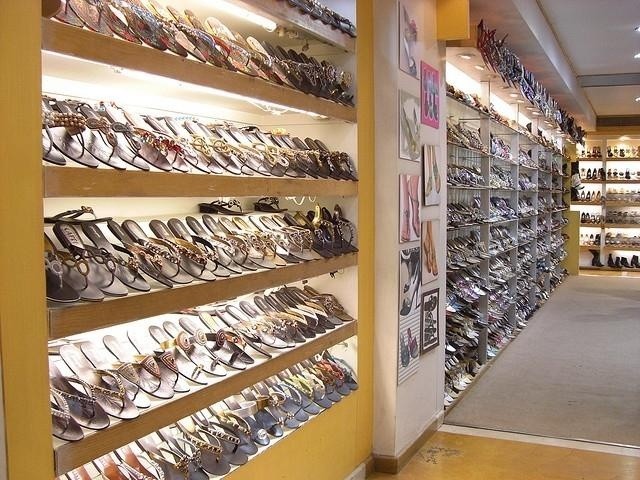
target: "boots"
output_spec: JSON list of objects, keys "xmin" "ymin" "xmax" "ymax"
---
[{"xmin": 589, "ymin": 250, "xmax": 601, "ymax": 266}]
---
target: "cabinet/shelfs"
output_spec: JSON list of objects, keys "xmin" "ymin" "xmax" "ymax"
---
[
  {"xmin": 570, "ymin": 136, "xmax": 640, "ymax": 276},
  {"xmin": 441, "ymin": 42, "xmax": 583, "ymax": 423},
  {"xmin": 37, "ymin": 0, "xmax": 364, "ymax": 479}
]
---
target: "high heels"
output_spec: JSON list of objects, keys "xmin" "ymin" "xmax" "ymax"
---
[{"xmin": 608, "ymin": 254, "xmax": 640, "ymax": 268}]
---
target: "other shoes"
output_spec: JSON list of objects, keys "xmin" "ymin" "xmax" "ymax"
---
[{"xmin": 445, "ymin": 20, "xmax": 585, "ymax": 408}]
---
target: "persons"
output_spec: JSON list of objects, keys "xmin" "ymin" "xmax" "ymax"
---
[
  {"xmin": 399, "ymin": 247, "xmax": 421, "ymax": 315},
  {"xmin": 424, "ymin": 144, "xmax": 441, "ymax": 197},
  {"xmin": 399, "ymin": 173, "xmax": 421, "ymax": 241},
  {"xmin": 423, "ymin": 220, "xmax": 439, "ymax": 276}
]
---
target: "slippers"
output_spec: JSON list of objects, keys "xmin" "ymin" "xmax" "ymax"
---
[
  {"xmin": 42, "ymin": 96, "xmax": 358, "ymax": 182},
  {"xmin": 43, "ymin": 197, "xmax": 359, "ymax": 302},
  {"xmin": 56, "ymin": 1, "xmax": 355, "ymax": 108},
  {"xmin": 400, "ymin": 37, "xmax": 441, "ymax": 367},
  {"xmin": 49, "ymin": 285, "xmax": 356, "ymax": 480}
]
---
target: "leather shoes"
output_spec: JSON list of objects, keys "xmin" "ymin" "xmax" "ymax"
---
[{"xmin": 581, "ymin": 146, "xmax": 640, "ymax": 247}]
